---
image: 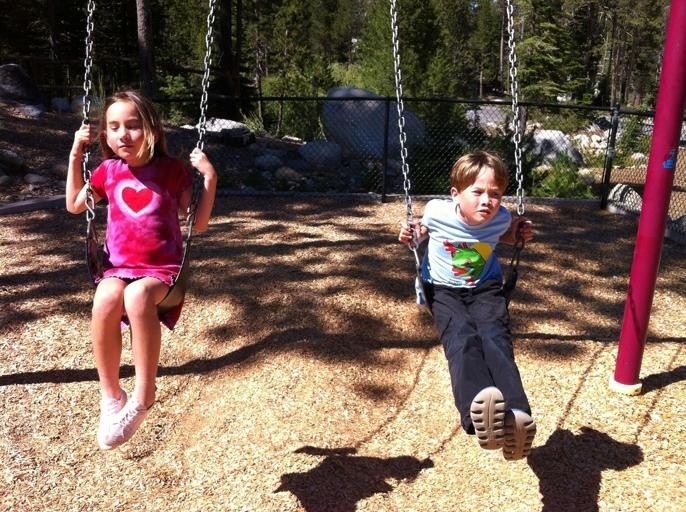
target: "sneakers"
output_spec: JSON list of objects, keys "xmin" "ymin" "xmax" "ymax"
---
[
  {"xmin": 470, "ymin": 385, "xmax": 505, "ymax": 452},
  {"xmin": 96, "ymin": 386, "xmax": 162, "ymax": 453},
  {"xmin": 504, "ymin": 409, "xmax": 536, "ymax": 461}
]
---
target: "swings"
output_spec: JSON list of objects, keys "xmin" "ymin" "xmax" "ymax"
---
[
  {"xmin": 83, "ymin": 0, "xmax": 217, "ymax": 311},
  {"xmin": 390, "ymin": 0, "xmax": 526, "ymax": 315}
]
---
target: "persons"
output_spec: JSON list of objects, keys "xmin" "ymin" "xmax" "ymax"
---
[
  {"xmin": 397, "ymin": 150, "xmax": 537, "ymax": 461},
  {"xmin": 65, "ymin": 88, "xmax": 218, "ymax": 451}
]
